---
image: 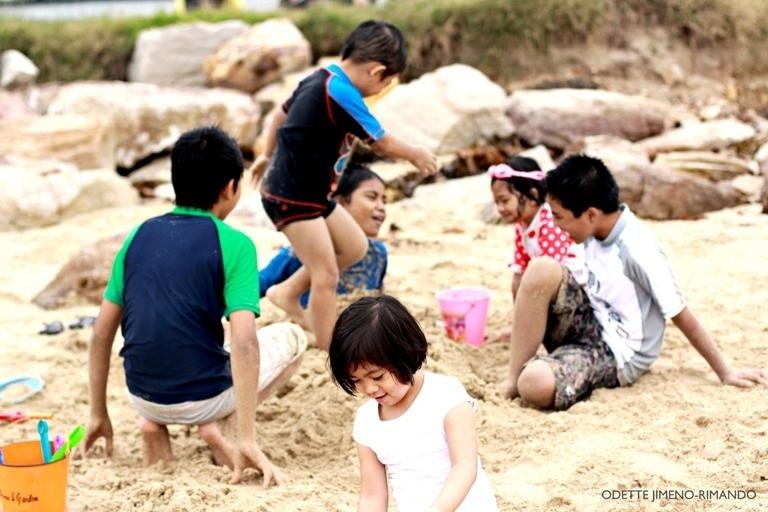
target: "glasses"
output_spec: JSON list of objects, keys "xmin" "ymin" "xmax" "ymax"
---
[{"xmin": 489, "ymin": 164, "xmax": 546, "ymax": 181}]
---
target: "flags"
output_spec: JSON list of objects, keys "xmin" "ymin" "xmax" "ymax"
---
[{"xmin": 1, "ymin": 409, "xmax": 84, "ymax": 465}]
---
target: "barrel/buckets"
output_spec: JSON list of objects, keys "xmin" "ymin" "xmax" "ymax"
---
[
  {"xmin": 0, "ymin": 440, "xmax": 70, "ymax": 512},
  {"xmin": 437, "ymin": 285, "xmax": 492, "ymax": 349}
]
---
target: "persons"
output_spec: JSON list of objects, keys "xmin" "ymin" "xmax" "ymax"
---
[
  {"xmin": 325, "ymin": 294, "xmax": 503, "ymax": 512},
  {"xmin": 249, "ymin": 19, "xmax": 442, "ymax": 351},
  {"xmin": 487, "ymin": 156, "xmax": 583, "ymax": 347},
  {"xmin": 258, "ymin": 164, "xmax": 389, "ymax": 310},
  {"xmin": 71, "ymin": 120, "xmax": 310, "ymax": 492},
  {"xmin": 492, "ymin": 153, "xmax": 768, "ymax": 412}
]
